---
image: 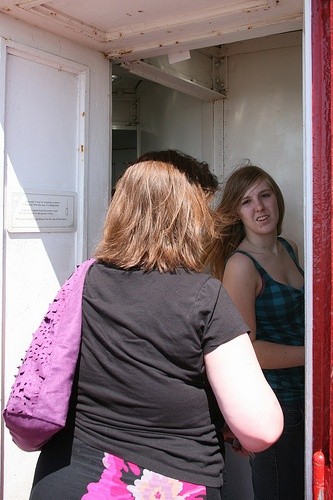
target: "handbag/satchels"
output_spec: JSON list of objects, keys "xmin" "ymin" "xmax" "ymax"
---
[{"xmin": 0, "ymin": 258, "xmax": 95, "ymax": 452}]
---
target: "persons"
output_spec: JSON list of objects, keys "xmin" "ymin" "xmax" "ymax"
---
[
  {"xmin": 27, "ymin": 149, "xmax": 282, "ymax": 500},
  {"xmin": 211, "ymin": 167, "xmax": 306, "ymax": 500}
]
